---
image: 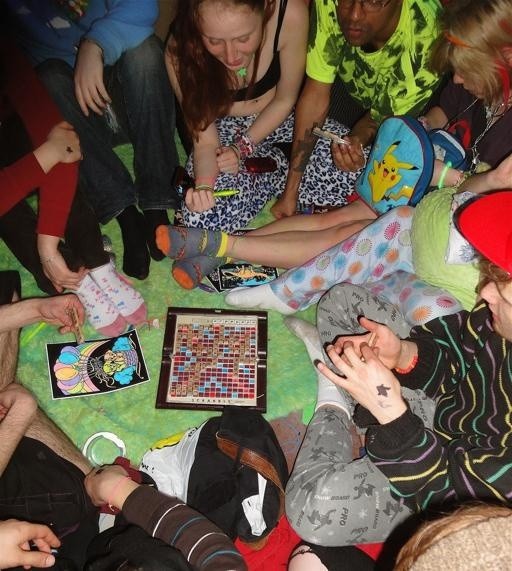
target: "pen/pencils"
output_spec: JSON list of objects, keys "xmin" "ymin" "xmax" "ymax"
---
[{"xmin": 212, "ymin": 191, "xmax": 239, "ymax": 197}]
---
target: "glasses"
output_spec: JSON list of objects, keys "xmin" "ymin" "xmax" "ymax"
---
[{"xmin": 330, "ymin": 0, "xmax": 392, "ymax": 11}]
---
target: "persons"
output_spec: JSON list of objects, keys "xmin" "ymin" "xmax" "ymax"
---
[{"xmin": 1, "ymin": 1, "xmax": 512, "ymax": 571}]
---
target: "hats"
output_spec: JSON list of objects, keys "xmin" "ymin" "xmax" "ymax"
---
[{"xmin": 455, "ymin": 187, "xmax": 512, "ymax": 279}]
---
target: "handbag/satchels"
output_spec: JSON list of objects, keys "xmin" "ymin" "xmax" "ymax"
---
[
  {"xmin": 351, "ymin": 112, "xmax": 478, "ymax": 223},
  {"xmin": 139, "ymin": 404, "xmax": 298, "ymax": 553}
]
